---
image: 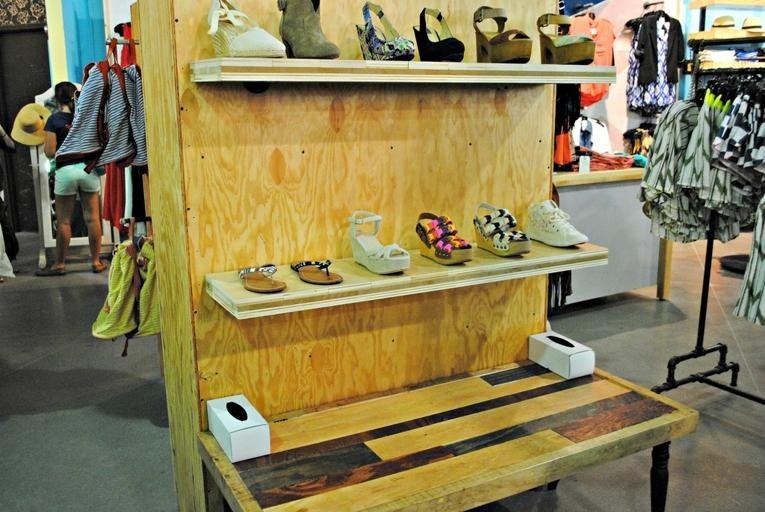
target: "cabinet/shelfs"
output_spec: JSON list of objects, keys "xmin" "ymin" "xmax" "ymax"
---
[
  {"xmin": 681, "ymin": 0, "xmax": 765, "ymax": 73},
  {"xmin": 187, "ymin": 53, "xmax": 617, "ymax": 326}
]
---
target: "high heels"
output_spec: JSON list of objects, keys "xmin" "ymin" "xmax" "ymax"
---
[
  {"xmin": 207, "ymin": 0, "xmax": 287, "ymax": 58},
  {"xmin": 473, "ymin": 202, "xmax": 531, "ymax": 257},
  {"xmin": 413, "ymin": 8, "xmax": 465, "ymax": 61},
  {"xmin": 473, "ymin": 7, "xmax": 532, "ymax": 63},
  {"xmin": 348, "ymin": 210, "xmax": 410, "ymax": 274},
  {"xmin": 537, "ymin": 13, "xmax": 595, "ymax": 65},
  {"xmin": 277, "ymin": 0, "xmax": 339, "ymax": 59},
  {"xmin": 356, "ymin": 2, "xmax": 415, "ymax": 61},
  {"xmin": 416, "ymin": 213, "xmax": 472, "ymax": 263}
]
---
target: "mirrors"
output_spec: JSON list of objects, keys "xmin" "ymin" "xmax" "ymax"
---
[{"xmin": 30, "ymin": 80, "xmax": 112, "ymax": 248}]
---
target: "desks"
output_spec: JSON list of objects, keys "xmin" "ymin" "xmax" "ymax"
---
[{"xmin": 195, "ymin": 355, "xmax": 701, "ymax": 512}]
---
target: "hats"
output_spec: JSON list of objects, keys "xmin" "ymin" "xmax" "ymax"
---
[
  {"xmin": 741, "ymin": 16, "xmax": 764, "ymax": 33},
  {"xmin": 12, "ymin": 103, "xmax": 53, "ymax": 146},
  {"xmin": 690, "ymin": 16, "xmax": 746, "ymax": 39}
]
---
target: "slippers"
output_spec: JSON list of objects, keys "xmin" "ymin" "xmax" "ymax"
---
[
  {"xmin": 239, "ymin": 263, "xmax": 287, "ymax": 293},
  {"xmin": 291, "ymin": 259, "xmax": 342, "ymax": 284}
]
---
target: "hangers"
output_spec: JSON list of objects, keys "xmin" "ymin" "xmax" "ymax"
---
[
  {"xmin": 624, "ymin": 114, "xmax": 658, "ymax": 138},
  {"xmin": 625, "ymin": 0, "xmax": 678, "ymax": 29},
  {"xmin": 692, "ymin": 69, "xmax": 765, "ymax": 100}
]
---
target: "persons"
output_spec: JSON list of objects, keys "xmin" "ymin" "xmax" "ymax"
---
[
  {"xmin": 34, "ymin": 80, "xmax": 110, "ymax": 275},
  {"xmin": 1, "ymin": 108, "xmax": 24, "ymax": 283}
]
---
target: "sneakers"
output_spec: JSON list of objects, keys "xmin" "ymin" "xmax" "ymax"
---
[
  {"xmin": 93, "ymin": 261, "xmax": 107, "ymax": 273},
  {"xmin": 525, "ymin": 200, "xmax": 588, "ymax": 247},
  {"xmin": 35, "ymin": 265, "xmax": 67, "ymax": 276}
]
